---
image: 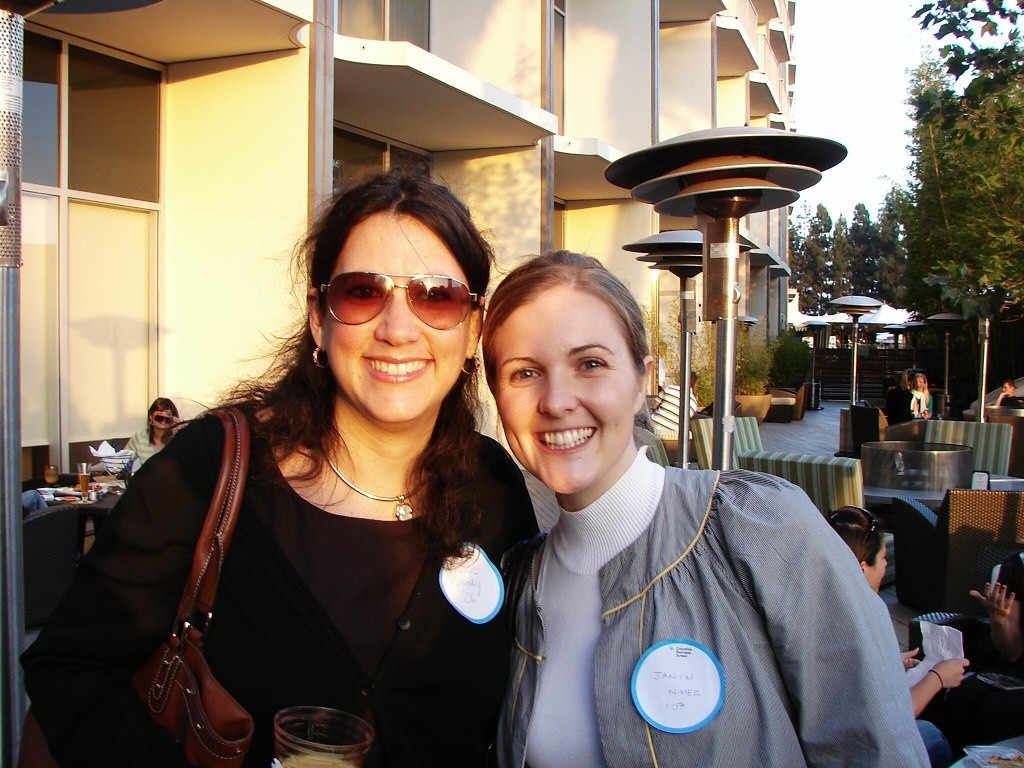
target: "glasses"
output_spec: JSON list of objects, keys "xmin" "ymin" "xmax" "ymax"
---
[
  {"xmin": 320, "ymin": 271, "xmax": 478, "ymax": 331},
  {"xmin": 831, "ymin": 505, "xmax": 881, "ymax": 546},
  {"xmin": 153, "ymin": 415, "xmax": 173, "ymax": 424}
]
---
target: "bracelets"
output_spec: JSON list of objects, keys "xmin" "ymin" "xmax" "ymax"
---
[{"xmin": 928, "ymin": 669, "xmax": 944, "ymax": 691}]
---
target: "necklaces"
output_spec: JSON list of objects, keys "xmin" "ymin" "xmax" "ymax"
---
[{"xmin": 318, "ymin": 426, "xmax": 436, "ymax": 522}]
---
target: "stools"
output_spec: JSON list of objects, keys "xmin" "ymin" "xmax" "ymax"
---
[
  {"xmin": 770, "ymin": 397, "xmax": 797, "ymax": 424},
  {"xmin": 648, "ymin": 426, "xmax": 692, "ymax": 464}
]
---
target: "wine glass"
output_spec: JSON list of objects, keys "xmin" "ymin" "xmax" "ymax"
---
[
  {"xmin": 936, "ymin": 414, "xmax": 944, "ymax": 421},
  {"xmin": 44, "ymin": 465, "xmax": 59, "ymax": 488}
]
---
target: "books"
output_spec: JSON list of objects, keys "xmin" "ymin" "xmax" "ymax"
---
[
  {"xmin": 976, "ymin": 672, "xmax": 1024, "ymax": 691},
  {"xmin": 88, "ymin": 440, "xmax": 142, "ymax": 495}
]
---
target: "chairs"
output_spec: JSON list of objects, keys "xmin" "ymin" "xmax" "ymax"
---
[
  {"xmin": 738, "ymin": 449, "xmax": 864, "ymax": 519},
  {"xmin": 770, "ymin": 382, "xmax": 809, "ymax": 420},
  {"xmin": 916, "ymin": 610, "xmax": 1024, "ymax": 744},
  {"xmin": 21, "ymin": 473, "xmax": 80, "ymax": 493},
  {"xmin": 765, "ymin": 422, "xmax": 771, "ymax": 423},
  {"xmin": 21, "ymin": 505, "xmax": 85, "ymax": 632},
  {"xmin": 924, "ymin": 419, "xmax": 1014, "ymax": 474},
  {"xmin": 691, "ymin": 416, "xmax": 763, "ymax": 470},
  {"xmin": 735, "ymin": 394, "xmax": 772, "ymax": 427},
  {"xmin": 646, "ymin": 395, "xmax": 659, "ymax": 408},
  {"xmin": 891, "ymin": 490, "xmax": 1024, "ymax": 615},
  {"xmin": 633, "ymin": 425, "xmax": 670, "ymax": 467},
  {"xmin": 849, "ymin": 404, "xmax": 886, "ymax": 442}
]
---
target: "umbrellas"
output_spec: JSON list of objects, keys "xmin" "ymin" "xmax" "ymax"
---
[{"xmin": 792, "ymin": 302, "xmax": 924, "ymax": 332}]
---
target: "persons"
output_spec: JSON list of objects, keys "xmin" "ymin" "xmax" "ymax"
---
[
  {"xmin": 883, "ymin": 369, "xmax": 934, "ymax": 427},
  {"xmin": 993, "ymin": 379, "xmax": 1024, "ymax": 410},
  {"xmin": 123, "ymin": 397, "xmax": 191, "ymax": 478},
  {"xmin": 966, "ymin": 549, "xmax": 1024, "ymax": 745},
  {"xmin": 21, "ymin": 490, "xmax": 49, "ymax": 520},
  {"xmin": 13, "ymin": 148, "xmax": 543, "ymax": 768},
  {"xmin": 480, "ymin": 246, "xmax": 932, "ymax": 768}
]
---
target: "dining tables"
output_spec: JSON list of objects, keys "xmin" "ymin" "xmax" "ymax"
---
[{"xmin": 43, "ymin": 480, "xmax": 128, "ymax": 519}]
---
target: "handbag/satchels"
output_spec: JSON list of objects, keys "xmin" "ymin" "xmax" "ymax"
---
[{"xmin": 17, "ymin": 408, "xmax": 254, "ymax": 767}]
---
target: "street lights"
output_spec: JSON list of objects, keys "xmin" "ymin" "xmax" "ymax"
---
[
  {"xmin": 883, "ymin": 325, "xmax": 907, "ymax": 371},
  {"xmin": 801, "ymin": 321, "xmax": 832, "ymax": 411},
  {"xmin": 904, "ymin": 322, "xmax": 928, "ymax": 370},
  {"xmin": 622, "ymin": 230, "xmax": 752, "ymax": 469},
  {"xmin": 926, "ymin": 313, "xmax": 964, "ymax": 420},
  {"xmin": 604, "ymin": 126, "xmax": 848, "ymax": 471},
  {"xmin": 826, "ymin": 295, "xmax": 882, "ymax": 458}
]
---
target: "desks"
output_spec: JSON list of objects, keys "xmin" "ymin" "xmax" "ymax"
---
[
  {"xmin": 887, "ymin": 419, "xmax": 926, "ymax": 441},
  {"xmin": 949, "ymin": 735, "xmax": 1024, "ymax": 768},
  {"xmin": 975, "ymin": 406, "xmax": 1024, "ymax": 478}
]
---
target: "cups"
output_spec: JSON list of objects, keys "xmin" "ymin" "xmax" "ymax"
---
[
  {"xmin": 79, "ymin": 474, "xmax": 90, "ymax": 504},
  {"xmin": 272, "ymin": 705, "xmax": 374, "ymax": 768}
]
